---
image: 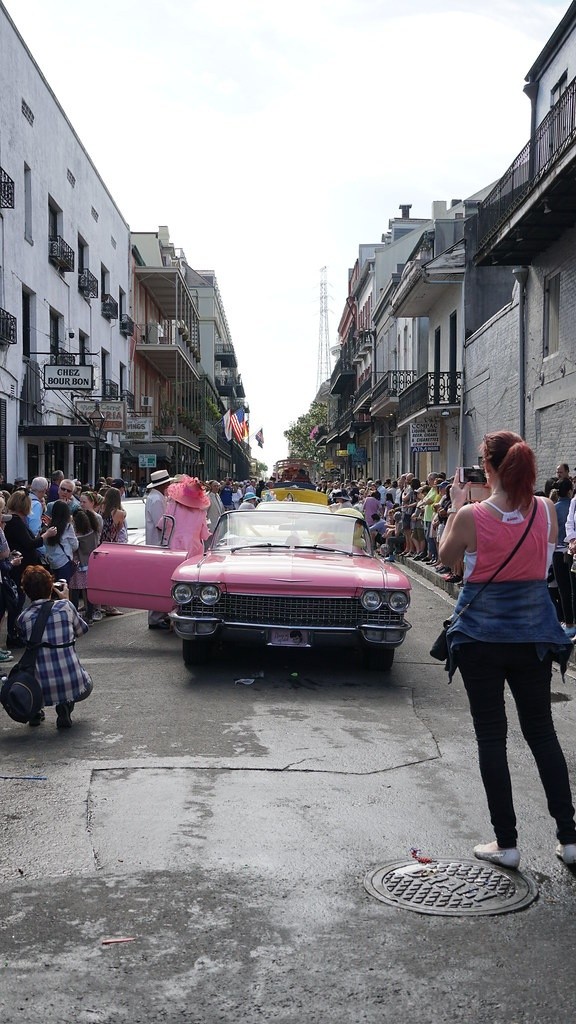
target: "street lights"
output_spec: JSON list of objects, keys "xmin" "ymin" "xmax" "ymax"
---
[{"xmin": 90, "ymin": 402, "xmax": 105, "ymax": 489}]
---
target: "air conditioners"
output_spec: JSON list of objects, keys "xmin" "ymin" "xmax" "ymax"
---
[{"xmin": 141, "ymin": 396, "xmax": 154, "ymax": 407}]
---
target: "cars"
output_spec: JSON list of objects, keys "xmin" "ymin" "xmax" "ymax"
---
[{"xmin": 118, "ymin": 498, "xmax": 146, "ymax": 545}]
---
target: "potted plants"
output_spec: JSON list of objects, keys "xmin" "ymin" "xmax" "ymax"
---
[
  {"xmin": 178, "ymin": 328, "xmax": 201, "ymax": 362},
  {"xmin": 156, "ymin": 399, "xmax": 202, "ymax": 436}
]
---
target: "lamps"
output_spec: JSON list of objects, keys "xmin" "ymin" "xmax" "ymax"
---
[
  {"xmin": 543, "ymin": 202, "xmax": 552, "ymax": 213},
  {"xmin": 515, "ymin": 231, "xmax": 523, "ymax": 241},
  {"xmin": 492, "ymin": 255, "xmax": 498, "ymax": 264}
]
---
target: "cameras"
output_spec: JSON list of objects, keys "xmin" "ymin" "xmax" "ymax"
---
[{"xmin": 51, "ymin": 579, "xmax": 68, "ymax": 600}]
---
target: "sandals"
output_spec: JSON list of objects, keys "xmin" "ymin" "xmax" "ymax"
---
[
  {"xmin": 105, "ymin": 607, "xmax": 123, "ymax": 615},
  {"xmin": 99, "ymin": 606, "xmax": 106, "ymax": 612}
]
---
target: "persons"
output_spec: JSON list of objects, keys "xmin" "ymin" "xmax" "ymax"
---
[
  {"xmin": 0, "ymin": 470, "xmax": 128, "ymax": 661},
  {"xmin": 439, "ymin": 431, "xmax": 576, "ymax": 869},
  {"xmin": 145, "ymin": 470, "xmax": 277, "ymax": 629},
  {"xmin": 127, "ymin": 480, "xmax": 142, "ymax": 498},
  {"xmin": 280, "ymin": 467, "xmax": 310, "ymax": 483},
  {"xmin": 17, "ymin": 565, "xmax": 93, "ymax": 728},
  {"xmin": 536, "ymin": 463, "xmax": 576, "ymax": 640},
  {"xmin": 316, "ymin": 472, "xmax": 463, "ymax": 587}
]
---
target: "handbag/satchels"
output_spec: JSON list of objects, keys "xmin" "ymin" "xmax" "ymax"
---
[
  {"xmin": 0, "ymin": 664, "xmax": 43, "ymax": 722},
  {"xmin": 428, "ymin": 613, "xmax": 454, "ymax": 662}
]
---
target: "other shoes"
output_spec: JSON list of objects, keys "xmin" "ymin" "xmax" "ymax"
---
[
  {"xmin": 555, "ymin": 844, "xmax": 576, "ymax": 863},
  {"xmin": 92, "ymin": 608, "xmax": 102, "ymax": 621},
  {"xmin": 148, "ymin": 617, "xmax": 174, "ymax": 630},
  {"xmin": 55, "ymin": 703, "xmax": 72, "ymax": 728},
  {"xmin": 76, "ymin": 604, "xmax": 85, "ymax": 610},
  {"xmin": 560, "ymin": 622, "xmax": 576, "ymax": 643},
  {"xmin": 0, "ymin": 653, "xmax": 14, "ymax": 662},
  {"xmin": 29, "ymin": 710, "xmax": 45, "ymax": 726},
  {"xmin": 377, "ymin": 544, "xmax": 464, "ymax": 589},
  {"xmin": 6, "ymin": 636, "xmax": 26, "ymax": 648},
  {"xmin": 0, "ymin": 647, "xmax": 11, "ymax": 656},
  {"xmin": 474, "ymin": 842, "xmax": 521, "ymax": 868}
]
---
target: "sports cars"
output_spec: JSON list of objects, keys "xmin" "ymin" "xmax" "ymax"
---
[{"xmin": 86, "ymin": 501, "xmax": 413, "ymax": 677}]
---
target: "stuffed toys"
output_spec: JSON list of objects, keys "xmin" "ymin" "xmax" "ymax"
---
[{"xmin": 316, "ymin": 508, "xmax": 366, "ymax": 547}]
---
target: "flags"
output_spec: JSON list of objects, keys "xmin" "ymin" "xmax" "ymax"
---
[
  {"xmin": 255, "ymin": 429, "xmax": 264, "ymax": 448},
  {"xmin": 223, "ymin": 409, "xmax": 248, "ymax": 443}
]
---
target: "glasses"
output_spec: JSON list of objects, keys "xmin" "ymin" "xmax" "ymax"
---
[{"xmin": 58, "ymin": 486, "xmax": 74, "ymax": 493}]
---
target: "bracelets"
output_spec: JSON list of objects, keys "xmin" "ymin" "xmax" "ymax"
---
[{"xmin": 447, "ymin": 508, "xmax": 457, "ymax": 514}]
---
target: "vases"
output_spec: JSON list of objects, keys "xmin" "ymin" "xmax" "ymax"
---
[{"xmin": 159, "ymin": 336, "xmax": 169, "ymax": 345}]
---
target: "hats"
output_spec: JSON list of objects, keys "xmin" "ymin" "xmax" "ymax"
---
[
  {"xmin": 2, "ymin": 514, "xmax": 12, "ymax": 522},
  {"xmin": 414, "ymin": 487, "xmax": 423, "ymax": 492},
  {"xmin": 243, "ymin": 492, "xmax": 257, "ymax": 502},
  {"xmin": 147, "ymin": 469, "xmax": 177, "ymax": 489},
  {"xmin": 15, "ymin": 476, "xmax": 28, "ymax": 482},
  {"xmin": 111, "ymin": 478, "xmax": 124, "ymax": 489},
  {"xmin": 437, "ymin": 480, "xmax": 449, "ymax": 487},
  {"xmin": 332, "ymin": 489, "xmax": 352, "ymax": 501},
  {"xmin": 167, "ymin": 477, "xmax": 211, "ymax": 509}
]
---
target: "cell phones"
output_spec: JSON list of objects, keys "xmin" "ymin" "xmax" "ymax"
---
[
  {"xmin": 459, "ymin": 467, "xmax": 487, "ymax": 484},
  {"xmin": 9, "ymin": 552, "xmax": 22, "ymax": 561}
]
